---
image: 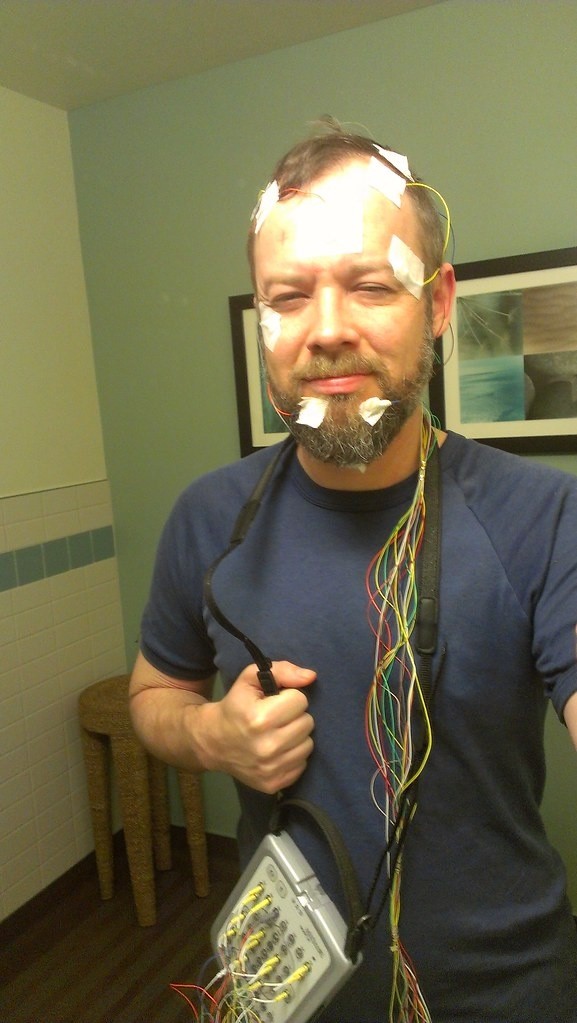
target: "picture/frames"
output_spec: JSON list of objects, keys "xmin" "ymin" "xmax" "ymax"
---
[
  {"xmin": 429, "ymin": 245, "xmax": 577, "ymax": 456},
  {"xmin": 228, "ymin": 292, "xmax": 291, "ymax": 458}
]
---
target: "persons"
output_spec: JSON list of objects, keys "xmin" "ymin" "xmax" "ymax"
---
[{"xmin": 127, "ymin": 134, "xmax": 577, "ymax": 1023}]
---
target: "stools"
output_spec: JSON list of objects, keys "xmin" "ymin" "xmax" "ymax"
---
[{"xmin": 79, "ymin": 672, "xmax": 210, "ymax": 928}]
